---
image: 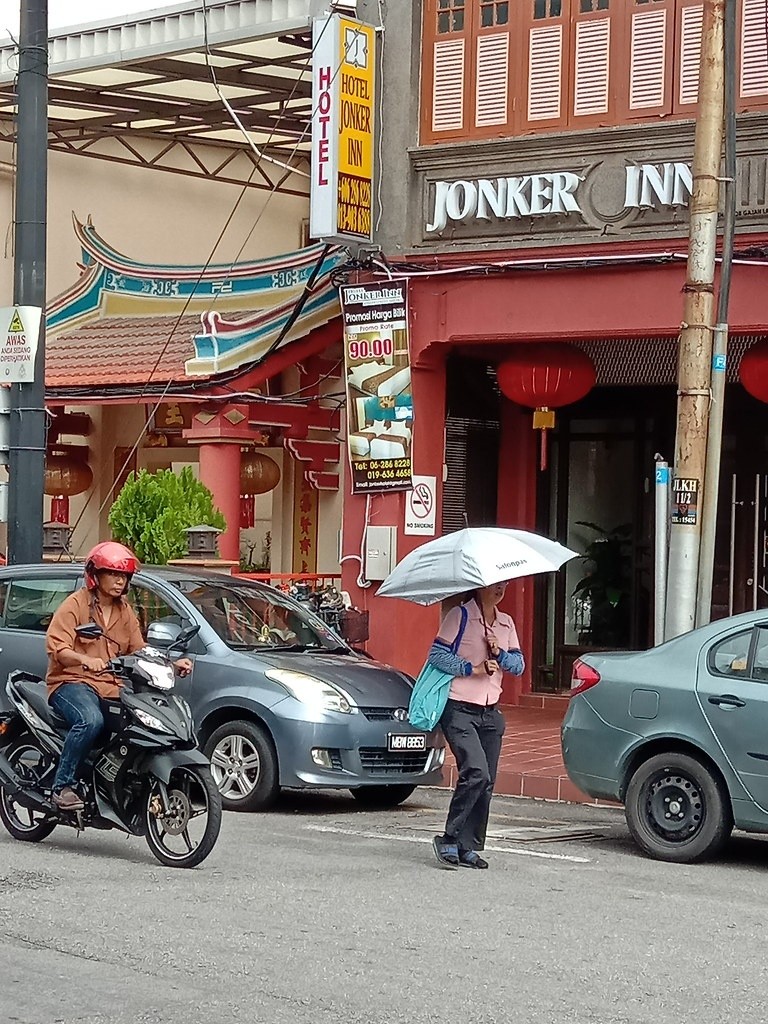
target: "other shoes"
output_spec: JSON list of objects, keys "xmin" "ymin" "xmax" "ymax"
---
[{"xmin": 53, "ymin": 787, "xmax": 85, "ymax": 810}]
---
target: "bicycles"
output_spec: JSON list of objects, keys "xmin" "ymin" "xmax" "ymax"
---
[{"xmin": 315, "ymin": 598, "xmax": 374, "ymax": 661}]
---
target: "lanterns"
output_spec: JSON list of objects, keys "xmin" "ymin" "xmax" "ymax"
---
[
  {"xmin": 495, "ymin": 337, "xmax": 597, "ymax": 470},
  {"xmin": 43, "ymin": 451, "xmax": 95, "ymax": 524},
  {"xmin": 737, "ymin": 333, "xmax": 768, "ymax": 404},
  {"xmin": 240, "ymin": 446, "xmax": 282, "ymax": 529}
]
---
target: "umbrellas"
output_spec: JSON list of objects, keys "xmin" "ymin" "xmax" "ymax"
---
[{"xmin": 372, "ymin": 509, "xmax": 583, "ymax": 676}]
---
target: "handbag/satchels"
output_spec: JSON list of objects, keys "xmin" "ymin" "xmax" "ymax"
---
[{"xmin": 407, "ymin": 605, "xmax": 469, "ymax": 731}]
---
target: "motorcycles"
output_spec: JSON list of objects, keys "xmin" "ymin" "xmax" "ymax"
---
[{"xmin": 0, "ymin": 625, "xmax": 222, "ymax": 869}]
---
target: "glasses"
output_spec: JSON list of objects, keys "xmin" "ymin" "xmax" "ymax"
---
[{"xmin": 487, "ymin": 580, "xmax": 509, "ymax": 587}]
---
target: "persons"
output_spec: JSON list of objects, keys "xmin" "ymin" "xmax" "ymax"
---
[
  {"xmin": 428, "ymin": 579, "xmax": 525, "ymax": 870},
  {"xmin": 44, "ymin": 541, "xmax": 193, "ymax": 810}
]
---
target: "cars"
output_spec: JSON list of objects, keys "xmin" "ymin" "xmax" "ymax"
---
[
  {"xmin": 560, "ymin": 608, "xmax": 768, "ymax": 864},
  {"xmin": 0, "ymin": 563, "xmax": 448, "ymax": 811}
]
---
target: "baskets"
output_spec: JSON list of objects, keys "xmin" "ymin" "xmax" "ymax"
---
[{"xmin": 339, "ymin": 609, "xmax": 369, "ymax": 643}]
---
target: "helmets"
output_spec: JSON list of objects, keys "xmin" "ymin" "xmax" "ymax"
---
[{"xmin": 83, "ymin": 542, "xmax": 142, "ymax": 595}]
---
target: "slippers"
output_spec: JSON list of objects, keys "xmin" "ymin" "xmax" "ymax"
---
[
  {"xmin": 433, "ymin": 835, "xmax": 460, "ymax": 869},
  {"xmin": 459, "ymin": 851, "xmax": 489, "ymax": 868}
]
